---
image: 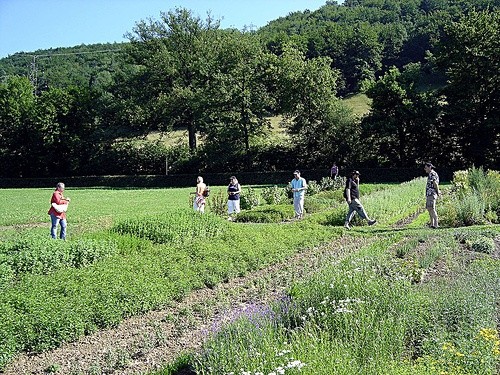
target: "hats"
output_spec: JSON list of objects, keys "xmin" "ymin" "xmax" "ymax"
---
[
  {"xmin": 294, "ymin": 170, "xmax": 300, "ymax": 175},
  {"xmin": 230, "ymin": 176, "xmax": 236, "ymax": 180},
  {"xmin": 352, "ymin": 170, "xmax": 360, "ymax": 175}
]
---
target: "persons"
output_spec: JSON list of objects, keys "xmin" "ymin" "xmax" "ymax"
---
[
  {"xmin": 291, "ymin": 170, "xmax": 307, "ymax": 217},
  {"xmin": 331, "ymin": 162, "xmax": 339, "ymax": 181},
  {"xmin": 48, "ymin": 182, "xmax": 70, "ymax": 239},
  {"xmin": 344, "ymin": 171, "xmax": 376, "ymax": 230},
  {"xmin": 190, "ymin": 176, "xmax": 205, "ymax": 213},
  {"xmin": 424, "ymin": 163, "xmax": 441, "ymax": 228},
  {"xmin": 227, "ymin": 176, "xmax": 241, "ymax": 221}
]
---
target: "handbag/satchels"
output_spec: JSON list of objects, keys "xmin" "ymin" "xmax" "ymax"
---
[
  {"xmin": 287, "ymin": 188, "xmax": 293, "ymax": 198},
  {"xmin": 51, "ymin": 200, "xmax": 69, "ymax": 212},
  {"xmin": 202, "ymin": 188, "xmax": 210, "ymax": 197}
]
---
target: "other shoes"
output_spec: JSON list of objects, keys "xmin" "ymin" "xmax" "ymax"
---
[
  {"xmin": 368, "ymin": 219, "xmax": 376, "ymax": 226},
  {"xmin": 344, "ymin": 224, "xmax": 351, "ymax": 231}
]
